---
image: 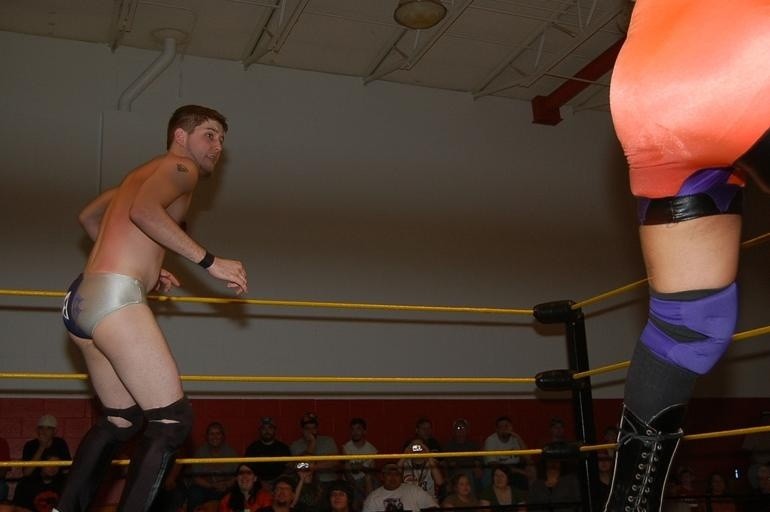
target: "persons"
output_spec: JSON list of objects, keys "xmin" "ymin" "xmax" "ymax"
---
[
  {"xmin": 53, "ymin": 104, "xmax": 249, "ymax": 512},
  {"xmin": 3, "ymin": 412, "xmax": 770, "ymax": 512},
  {"xmin": 602, "ymin": 0, "xmax": 770, "ymax": 509}
]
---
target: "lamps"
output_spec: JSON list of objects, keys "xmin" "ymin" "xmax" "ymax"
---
[{"xmin": 393, "ymin": 0, "xmax": 447, "ymax": 29}]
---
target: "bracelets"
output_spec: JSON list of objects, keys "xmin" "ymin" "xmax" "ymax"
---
[{"xmin": 197, "ymin": 252, "xmax": 215, "ymax": 270}]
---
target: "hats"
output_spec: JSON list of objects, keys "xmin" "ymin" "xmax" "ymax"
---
[
  {"xmin": 37, "ymin": 415, "xmax": 56, "ymax": 428},
  {"xmin": 257, "ymin": 417, "xmax": 276, "ymax": 429},
  {"xmin": 382, "ymin": 463, "xmax": 398, "ymax": 472},
  {"xmin": 301, "ymin": 412, "xmax": 317, "ymax": 426}
]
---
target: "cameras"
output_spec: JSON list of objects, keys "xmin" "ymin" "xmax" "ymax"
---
[
  {"xmin": 294, "ymin": 462, "xmax": 310, "ymax": 470},
  {"xmin": 411, "ymin": 442, "xmax": 423, "ymax": 453},
  {"xmin": 455, "ymin": 422, "xmax": 465, "ymax": 432}
]
